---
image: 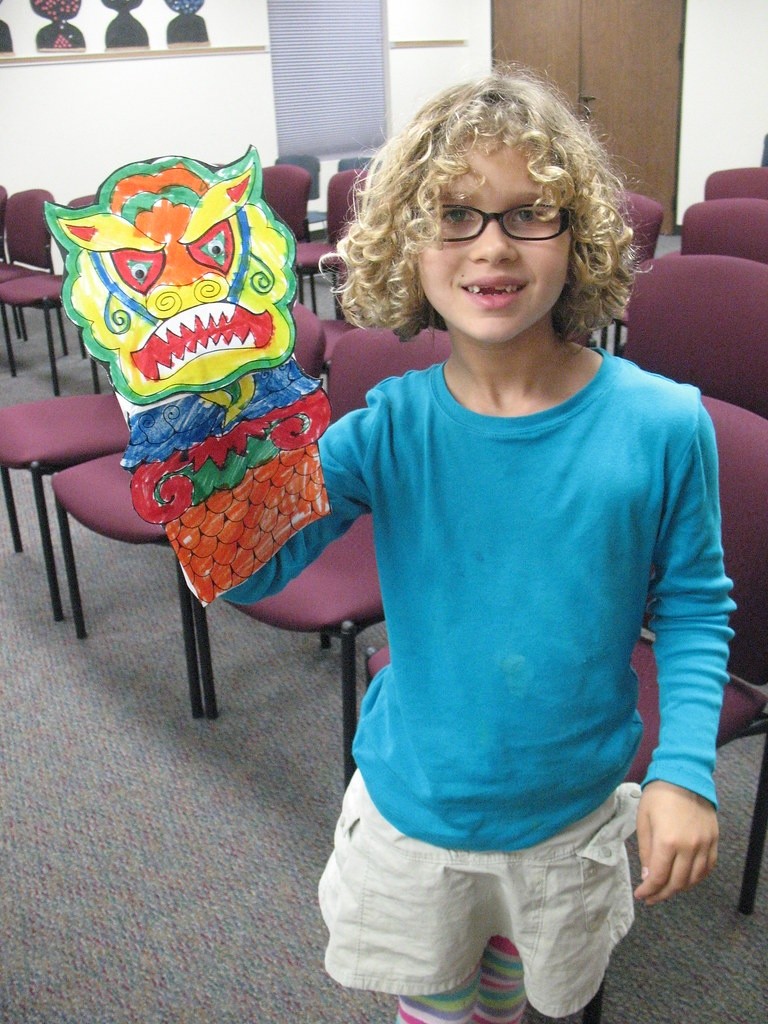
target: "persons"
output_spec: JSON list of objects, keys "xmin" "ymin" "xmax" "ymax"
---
[{"xmin": 218, "ymin": 79, "xmax": 738, "ymax": 1023}]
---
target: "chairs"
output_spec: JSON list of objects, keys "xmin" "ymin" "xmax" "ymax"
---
[
  {"xmin": 703, "ymin": 166, "xmax": 768, "ymax": 201},
  {"xmin": 613, "ymin": 195, "xmax": 768, "ymax": 356},
  {"xmin": 0, "ymin": 393, "xmax": 133, "ymax": 625},
  {"xmin": 595, "ymin": 187, "xmax": 664, "ymax": 353},
  {"xmin": 356, "ymin": 392, "xmax": 768, "ymax": 915},
  {"xmin": 292, "ymin": 169, "xmax": 376, "ymax": 321},
  {"xmin": 260, "ymin": 166, "xmax": 317, "ymax": 314},
  {"xmin": 622, "ymin": 251, "xmax": 768, "ymax": 418},
  {"xmin": 51, "ymin": 300, "xmax": 333, "ymax": 720},
  {"xmin": 184, "ymin": 321, "xmax": 451, "ymax": 792},
  {"xmin": 0, "ymin": 193, "xmax": 96, "ymax": 396},
  {"xmin": 0, "ymin": 188, "xmax": 57, "ymax": 341},
  {"xmin": 0, "ymin": 185, "xmax": 22, "ymax": 340}
]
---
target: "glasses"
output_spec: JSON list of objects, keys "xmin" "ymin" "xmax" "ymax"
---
[{"xmin": 415, "ymin": 205, "xmax": 571, "ymax": 240}]
